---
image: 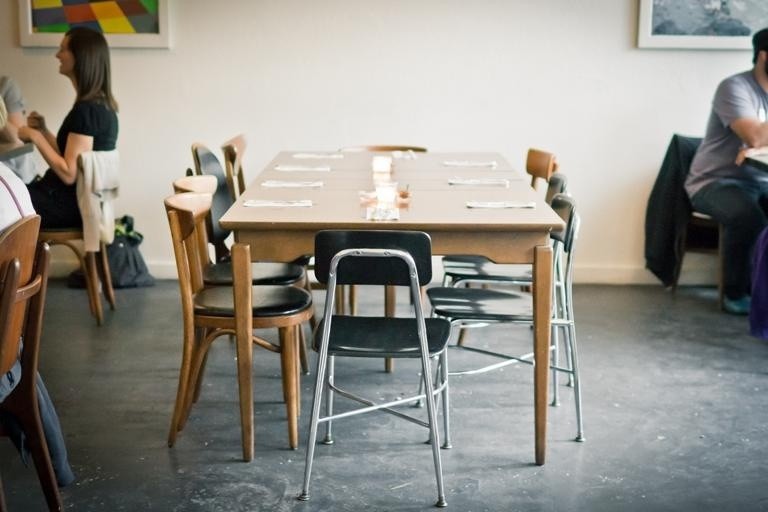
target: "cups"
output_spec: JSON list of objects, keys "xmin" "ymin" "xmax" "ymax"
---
[
  {"xmin": 396, "ymin": 183, "xmax": 415, "ymax": 210},
  {"xmin": 357, "ymin": 188, "xmax": 378, "ymax": 220}
]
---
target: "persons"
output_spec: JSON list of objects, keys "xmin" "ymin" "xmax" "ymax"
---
[
  {"xmin": 681, "ymin": 24, "xmax": 768, "ymax": 315},
  {"xmin": 16, "ymin": 25, "xmax": 121, "ymax": 233},
  {"xmin": 0, "ymin": 74, "xmax": 24, "ymax": 151},
  {"xmin": 0, "ymin": 159, "xmax": 36, "ymax": 238}
]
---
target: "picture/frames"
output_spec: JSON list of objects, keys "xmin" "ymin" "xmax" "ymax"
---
[
  {"xmin": 636, "ymin": 0, "xmax": 766, "ymax": 50},
  {"xmin": 17, "ymin": 0, "xmax": 170, "ymax": 50}
]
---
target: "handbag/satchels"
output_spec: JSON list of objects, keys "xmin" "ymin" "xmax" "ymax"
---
[{"xmin": 66, "ymin": 214, "xmax": 157, "ymax": 288}]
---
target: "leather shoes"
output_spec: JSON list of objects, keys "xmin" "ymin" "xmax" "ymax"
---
[{"xmin": 721, "ymin": 291, "xmax": 752, "ymax": 315}]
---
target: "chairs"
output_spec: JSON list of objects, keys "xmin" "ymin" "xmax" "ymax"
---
[
  {"xmin": 162, "ymin": 192, "xmax": 313, "ymax": 464},
  {"xmin": 38, "ymin": 147, "xmax": 120, "ymax": 327},
  {"xmin": 427, "ymin": 173, "xmax": 577, "ymax": 390},
  {"xmin": 0, "ymin": 213, "xmax": 66, "ymax": 512},
  {"xmin": 669, "ymin": 135, "xmax": 725, "ymax": 304},
  {"xmin": 441, "ymin": 147, "xmax": 558, "ymax": 290},
  {"xmin": 415, "ymin": 196, "xmax": 585, "ymax": 446},
  {"xmin": 183, "ymin": 141, "xmax": 316, "ymax": 335},
  {"xmin": 173, "ymin": 173, "xmax": 311, "ymax": 404},
  {"xmin": 366, "ymin": 143, "xmax": 424, "ymax": 154},
  {"xmin": 298, "ymin": 226, "xmax": 452, "ymax": 512},
  {"xmin": 222, "ymin": 134, "xmax": 246, "ymax": 202}
]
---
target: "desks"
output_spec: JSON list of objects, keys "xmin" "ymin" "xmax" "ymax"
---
[
  {"xmin": 219, "ymin": 147, "xmax": 567, "ymax": 465},
  {"xmin": 738, "ymin": 145, "xmax": 768, "ymax": 172}
]
